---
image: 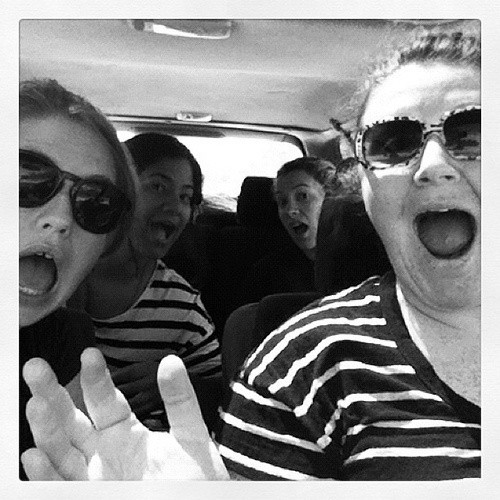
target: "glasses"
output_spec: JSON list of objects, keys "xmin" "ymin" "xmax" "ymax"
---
[
  {"xmin": 20, "ymin": 147, "xmax": 132, "ymax": 235},
  {"xmin": 355, "ymin": 104, "xmax": 482, "ymax": 173}
]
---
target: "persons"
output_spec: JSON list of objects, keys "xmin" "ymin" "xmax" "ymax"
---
[
  {"xmin": 273, "ymin": 156, "xmax": 337, "ymax": 293},
  {"xmin": 61, "ymin": 131, "xmax": 222, "ymax": 432},
  {"xmin": 19, "ymin": 27, "xmax": 482, "ymax": 481},
  {"xmin": 19, "ymin": 78, "xmax": 144, "ymax": 480}
]
